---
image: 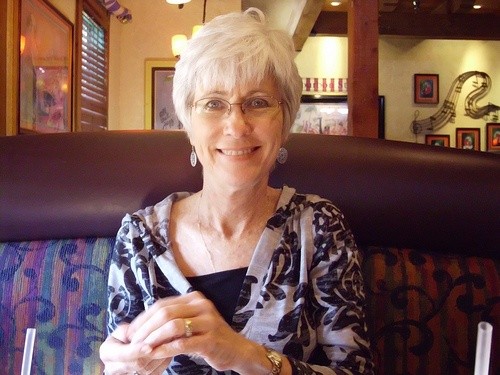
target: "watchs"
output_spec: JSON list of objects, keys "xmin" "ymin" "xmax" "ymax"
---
[{"xmin": 265, "ymin": 347, "xmax": 283, "ymax": 375}]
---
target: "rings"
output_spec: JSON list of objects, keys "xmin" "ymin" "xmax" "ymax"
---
[
  {"xmin": 133, "ymin": 370, "xmax": 140, "ymax": 375},
  {"xmin": 185, "ymin": 318, "xmax": 193, "ymax": 337}
]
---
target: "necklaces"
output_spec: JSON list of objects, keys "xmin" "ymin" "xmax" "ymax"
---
[{"xmin": 197, "ymin": 190, "xmax": 270, "ymax": 272}]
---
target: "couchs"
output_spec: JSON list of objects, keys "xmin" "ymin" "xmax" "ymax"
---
[{"xmin": 0, "ymin": 130, "xmax": 500, "ymax": 375}]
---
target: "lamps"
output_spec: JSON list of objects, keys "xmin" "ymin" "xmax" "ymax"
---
[
  {"xmin": 173, "ymin": 0, "xmax": 208, "ymax": 58},
  {"xmin": 166, "ymin": 0, "xmax": 192, "ymax": 5}
]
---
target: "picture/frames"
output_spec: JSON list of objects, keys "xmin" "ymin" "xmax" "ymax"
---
[
  {"xmin": 143, "ymin": 58, "xmax": 185, "ymax": 130},
  {"xmin": 455, "ymin": 126, "xmax": 481, "ymax": 151},
  {"xmin": 413, "ymin": 71, "xmax": 441, "ymax": 105},
  {"xmin": 424, "ymin": 133, "xmax": 451, "ymax": 147},
  {"xmin": 486, "ymin": 122, "xmax": 500, "ymax": 152},
  {"xmin": 289, "ymin": 93, "xmax": 386, "ymax": 139},
  {"xmin": 31, "ymin": 65, "xmax": 68, "ymax": 131},
  {"xmin": 11, "ymin": 0, "xmax": 75, "ymax": 137}
]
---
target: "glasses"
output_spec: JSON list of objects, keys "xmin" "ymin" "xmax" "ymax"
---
[{"xmin": 188, "ymin": 95, "xmax": 287, "ymax": 118}]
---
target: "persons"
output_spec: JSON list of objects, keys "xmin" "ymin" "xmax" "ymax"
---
[{"xmin": 100, "ymin": 8, "xmax": 375, "ymax": 375}]
